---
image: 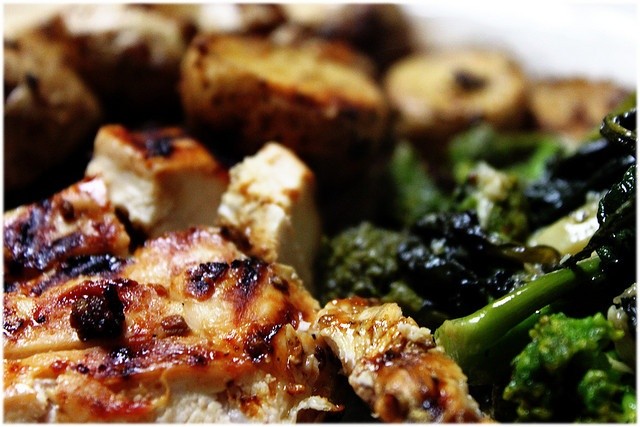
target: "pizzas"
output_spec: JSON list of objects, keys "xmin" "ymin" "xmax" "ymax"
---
[{"xmin": 2, "ymin": 123, "xmax": 493, "ymax": 421}]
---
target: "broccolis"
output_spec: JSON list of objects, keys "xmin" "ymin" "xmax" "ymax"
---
[{"xmin": 315, "ymin": 110, "xmax": 636, "ymax": 423}]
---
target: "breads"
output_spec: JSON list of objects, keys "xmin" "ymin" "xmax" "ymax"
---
[{"xmin": 3, "ymin": 4, "xmax": 635, "ymax": 194}]
